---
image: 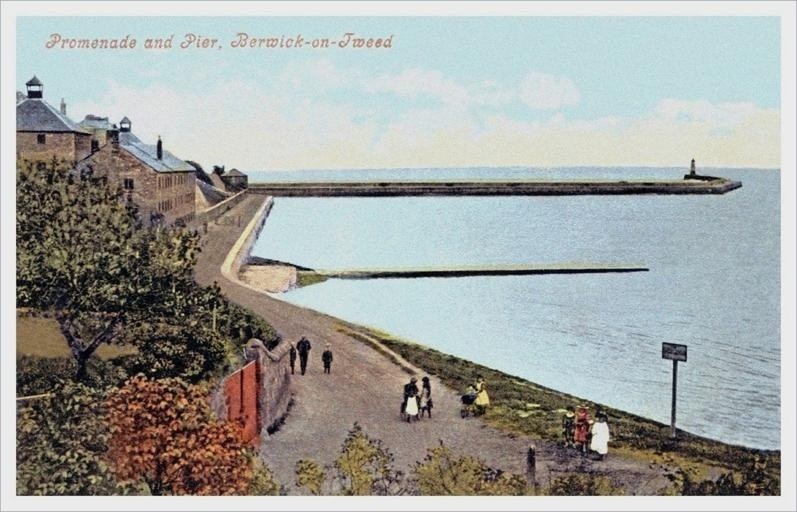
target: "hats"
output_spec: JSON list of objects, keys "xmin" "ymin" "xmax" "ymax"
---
[{"xmin": 576, "ymin": 403, "xmax": 590, "ymax": 410}]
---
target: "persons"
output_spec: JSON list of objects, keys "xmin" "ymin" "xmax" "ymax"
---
[
  {"xmin": 321, "ymin": 341, "xmax": 333, "ymax": 374},
  {"xmin": 589, "ymin": 409, "xmax": 609, "ymax": 462},
  {"xmin": 561, "ymin": 404, "xmax": 577, "ymax": 449},
  {"xmin": 459, "ymin": 384, "xmax": 480, "ymax": 420},
  {"xmin": 573, "ymin": 403, "xmax": 589, "ymax": 452},
  {"xmin": 399, "ymin": 376, "xmax": 420, "ymax": 423},
  {"xmin": 288, "ymin": 341, "xmax": 296, "ymax": 375},
  {"xmin": 416, "ymin": 377, "xmax": 433, "ymax": 419},
  {"xmin": 469, "ymin": 373, "xmax": 491, "ymax": 419},
  {"xmin": 294, "ymin": 333, "xmax": 312, "ymax": 376}
]
